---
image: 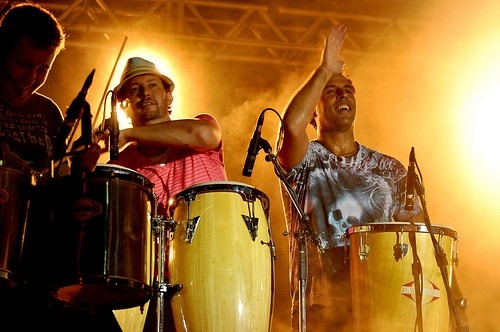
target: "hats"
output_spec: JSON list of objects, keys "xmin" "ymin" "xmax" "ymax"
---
[{"xmin": 113, "ymin": 57, "xmax": 176, "ymax": 99}]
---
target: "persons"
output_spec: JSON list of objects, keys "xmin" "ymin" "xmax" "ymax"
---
[
  {"xmin": 92, "ymin": 57, "xmax": 228, "ymax": 332},
  {"xmin": 0, "ymin": 3, "xmax": 100, "ymax": 174},
  {"xmin": 277, "ymin": 23, "xmax": 425, "ymax": 332}
]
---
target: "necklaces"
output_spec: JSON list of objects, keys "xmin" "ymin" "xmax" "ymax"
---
[{"xmin": 337, "ymin": 148, "xmax": 358, "ymax": 161}]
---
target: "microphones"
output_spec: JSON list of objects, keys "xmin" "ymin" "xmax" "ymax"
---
[
  {"xmin": 405, "ymin": 147, "xmax": 416, "ymax": 211},
  {"xmin": 110, "ymin": 92, "xmax": 118, "ymax": 160},
  {"xmin": 52, "ymin": 69, "xmax": 96, "ymax": 147},
  {"xmin": 241, "ymin": 111, "xmax": 264, "ymax": 177}
]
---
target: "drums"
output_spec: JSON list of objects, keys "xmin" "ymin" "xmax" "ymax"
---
[
  {"xmin": 0, "ymin": 158, "xmax": 43, "ymax": 283},
  {"xmin": 344, "ymin": 221, "xmax": 459, "ymax": 332},
  {"xmin": 166, "ymin": 181, "xmax": 275, "ymax": 331},
  {"xmin": 44, "ymin": 165, "xmax": 156, "ymax": 310}
]
